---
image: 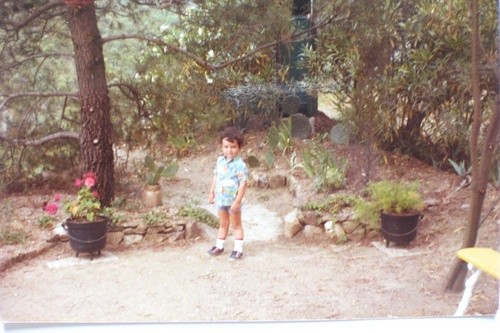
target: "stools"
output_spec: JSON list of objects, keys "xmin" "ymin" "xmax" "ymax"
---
[{"xmin": 453, "ymin": 247, "xmax": 499, "ymax": 317}]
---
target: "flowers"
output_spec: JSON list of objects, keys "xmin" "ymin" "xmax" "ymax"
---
[{"xmin": 41, "ymin": 173, "xmax": 102, "ymax": 222}]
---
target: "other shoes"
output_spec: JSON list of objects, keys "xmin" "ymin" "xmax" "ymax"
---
[
  {"xmin": 228, "ymin": 250, "xmax": 244, "ymax": 260},
  {"xmin": 206, "ymin": 246, "xmax": 225, "ymax": 257}
]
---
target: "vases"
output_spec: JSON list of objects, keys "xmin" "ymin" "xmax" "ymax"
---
[{"xmin": 62, "ymin": 215, "xmax": 108, "ymax": 260}]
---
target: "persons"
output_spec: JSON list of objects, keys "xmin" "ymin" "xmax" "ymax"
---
[{"xmin": 208, "ymin": 129, "xmax": 250, "ymax": 262}]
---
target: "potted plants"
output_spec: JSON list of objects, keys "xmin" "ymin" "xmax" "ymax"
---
[
  {"xmin": 356, "ymin": 181, "xmax": 423, "ymax": 247},
  {"xmin": 135, "ymin": 156, "xmax": 179, "ymax": 207}
]
